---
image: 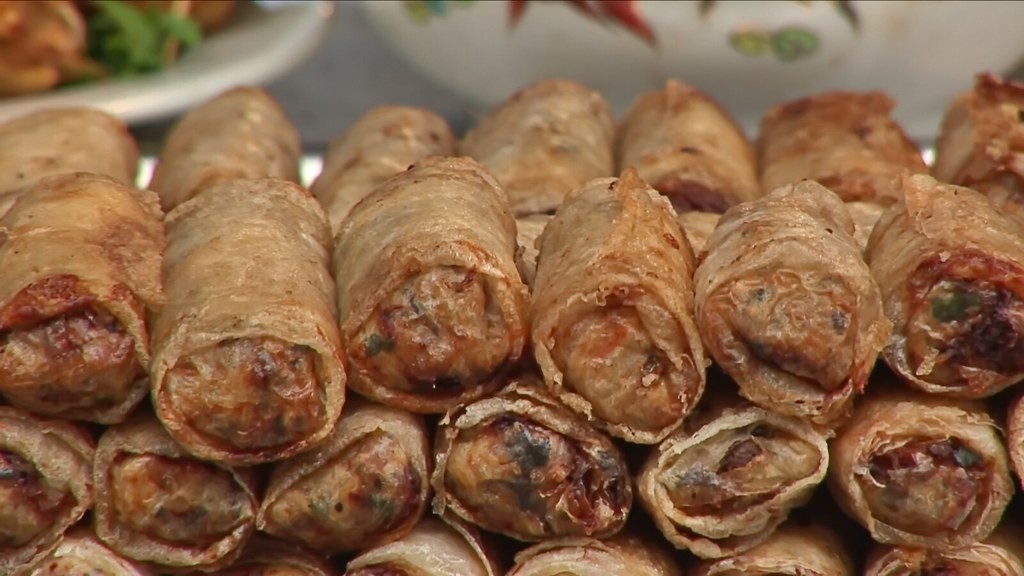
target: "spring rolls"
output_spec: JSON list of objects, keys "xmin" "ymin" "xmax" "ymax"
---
[{"xmin": 0, "ymin": 82, "xmax": 1024, "ymax": 576}]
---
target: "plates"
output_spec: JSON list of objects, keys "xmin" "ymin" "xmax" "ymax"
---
[{"xmin": 1, "ymin": 0, "xmax": 331, "ymax": 131}]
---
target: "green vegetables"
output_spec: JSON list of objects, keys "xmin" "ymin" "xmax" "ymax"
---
[{"xmin": 53, "ymin": 0, "xmax": 209, "ymax": 91}]
